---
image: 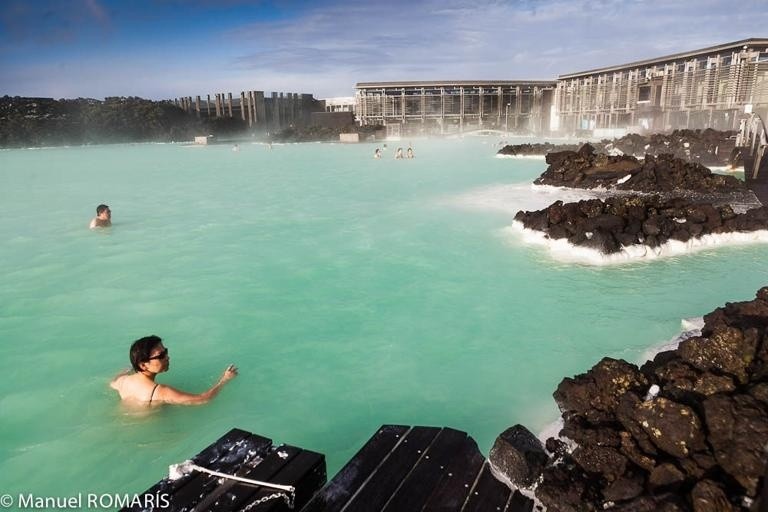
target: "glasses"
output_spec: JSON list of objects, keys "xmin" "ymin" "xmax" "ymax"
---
[{"xmin": 148, "ymin": 348, "xmax": 167, "ymax": 360}]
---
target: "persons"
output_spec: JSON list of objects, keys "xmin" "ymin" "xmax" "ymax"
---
[
  {"xmin": 231, "ymin": 142, "xmax": 239, "ymax": 151},
  {"xmin": 110, "ymin": 336, "xmax": 239, "ymax": 419},
  {"xmin": 373, "ymin": 142, "xmax": 417, "ymax": 160},
  {"xmin": 88, "ymin": 204, "xmax": 112, "ymax": 230},
  {"xmin": 265, "ymin": 141, "xmax": 275, "ymax": 153}
]
